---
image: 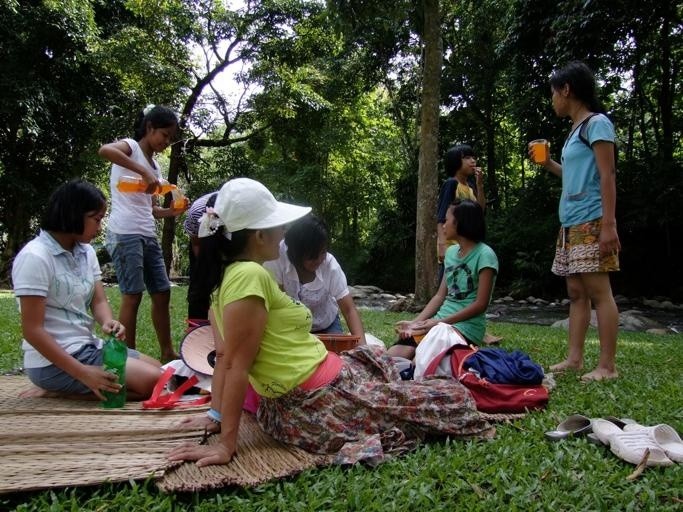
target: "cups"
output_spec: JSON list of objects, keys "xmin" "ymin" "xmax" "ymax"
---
[
  {"xmin": 172, "ymin": 188, "xmax": 186, "ymax": 209},
  {"xmin": 531, "ymin": 140, "xmax": 548, "ymax": 163}
]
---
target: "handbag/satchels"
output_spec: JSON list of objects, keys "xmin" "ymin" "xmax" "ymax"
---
[{"xmin": 142, "ymin": 359, "xmax": 213, "ymax": 411}]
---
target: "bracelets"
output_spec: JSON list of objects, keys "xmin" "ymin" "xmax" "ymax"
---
[{"xmin": 205, "ymin": 409, "xmax": 222, "ymax": 424}]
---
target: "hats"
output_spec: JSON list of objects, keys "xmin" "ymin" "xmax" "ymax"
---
[
  {"xmin": 179, "ymin": 324, "xmax": 218, "ymax": 376},
  {"xmin": 210, "ymin": 177, "xmax": 313, "ymax": 232}
]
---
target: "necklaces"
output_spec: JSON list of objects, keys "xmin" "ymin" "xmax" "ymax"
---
[{"xmin": 561, "ymin": 115, "xmax": 586, "ymax": 162}]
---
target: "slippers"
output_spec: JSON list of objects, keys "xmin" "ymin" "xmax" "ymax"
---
[
  {"xmin": 587, "ymin": 415, "xmax": 636, "ymax": 446},
  {"xmin": 622, "ymin": 423, "xmax": 683, "ymax": 463},
  {"xmin": 544, "ymin": 413, "xmax": 593, "ymax": 442},
  {"xmin": 593, "ymin": 419, "xmax": 674, "ymax": 467}
]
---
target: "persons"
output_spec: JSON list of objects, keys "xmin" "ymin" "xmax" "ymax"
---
[
  {"xmin": 436, "ymin": 145, "xmax": 504, "ymax": 345},
  {"xmin": 383, "ymin": 198, "xmax": 500, "ymax": 359},
  {"xmin": 527, "ymin": 61, "xmax": 621, "ymax": 380},
  {"xmin": 185, "ymin": 190, "xmax": 218, "ymax": 332},
  {"xmin": 96, "ymin": 103, "xmax": 191, "ymax": 362},
  {"xmin": 265, "ymin": 214, "xmax": 368, "ymax": 353},
  {"xmin": 12, "ymin": 182, "xmax": 165, "ymax": 401},
  {"xmin": 167, "ymin": 178, "xmax": 495, "ymax": 467}
]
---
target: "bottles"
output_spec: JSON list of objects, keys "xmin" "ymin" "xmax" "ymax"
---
[
  {"xmin": 117, "ymin": 174, "xmax": 177, "ymax": 195},
  {"xmin": 99, "ymin": 330, "xmax": 128, "ymax": 409}
]
